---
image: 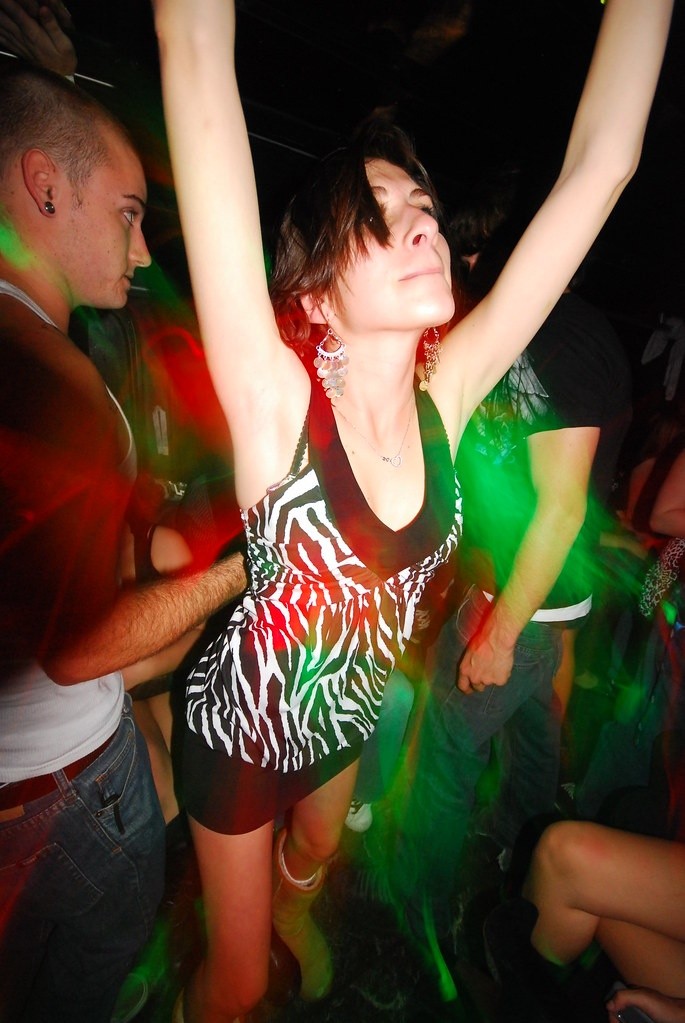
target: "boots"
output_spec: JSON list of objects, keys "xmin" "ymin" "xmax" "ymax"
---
[{"xmin": 273, "ymin": 829, "xmax": 334, "ymax": 1004}]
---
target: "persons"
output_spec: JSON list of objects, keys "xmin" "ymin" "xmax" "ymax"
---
[
  {"xmin": 150, "ymin": 0, "xmax": 671, "ymax": 1023},
  {"xmin": 99, "ymin": 201, "xmax": 685, "ymax": 1023},
  {"xmin": 0, "ymin": 64, "xmax": 250, "ymax": 1023},
  {"xmin": 0, "ymin": 0, "xmax": 80, "ymax": 83}
]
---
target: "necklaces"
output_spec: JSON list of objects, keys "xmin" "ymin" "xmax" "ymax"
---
[{"xmin": 329, "ymin": 399, "xmax": 417, "ymax": 467}]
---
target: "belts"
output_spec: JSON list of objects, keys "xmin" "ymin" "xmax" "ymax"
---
[{"xmin": 1, "ymin": 728, "xmax": 117, "ymax": 829}]
